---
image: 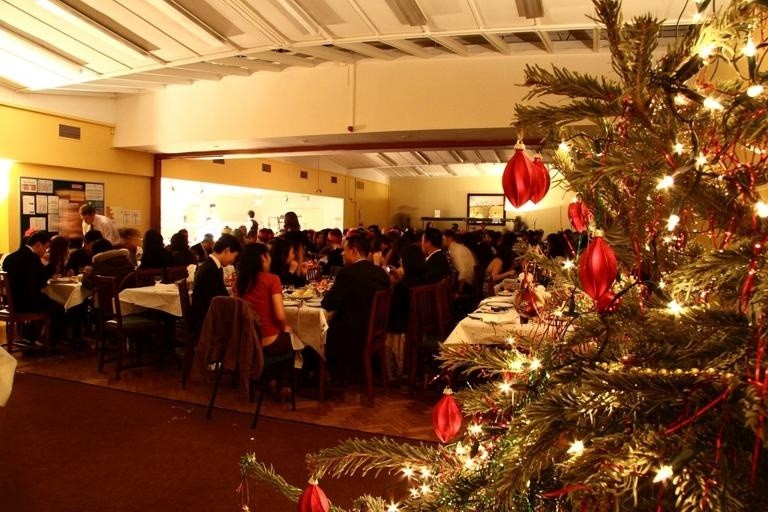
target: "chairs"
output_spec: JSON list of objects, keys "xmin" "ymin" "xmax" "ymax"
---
[
  {"xmin": 363, "ymin": 291, "xmax": 392, "ymax": 408},
  {"xmin": 404, "ymin": 286, "xmax": 442, "ymax": 386},
  {"xmin": 0, "ymin": 266, "xmax": 49, "ymax": 359},
  {"xmin": 96, "ymin": 275, "xmax": 156, "ymax": 376},
  {"xmin": 176, "ymin": 278, "xmax": 245, "ymax": 390},
  {"xmin": 206, "ymin": 295, "xmax": 296, "ymax": 431}
]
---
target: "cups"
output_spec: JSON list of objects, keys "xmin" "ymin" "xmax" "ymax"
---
[{"xmin": 154, "ymin": 276, "xmax": 162, "ymax": 286}]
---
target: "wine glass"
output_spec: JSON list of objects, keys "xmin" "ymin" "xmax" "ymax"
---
[{"xmin": 316, "ymin": 282, "xmax": 325, "ymax": 299}]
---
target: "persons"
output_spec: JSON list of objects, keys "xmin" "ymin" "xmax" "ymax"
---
[{"xmin": 0, "ymin": 201, "xmax": 587, "ymax": 399}]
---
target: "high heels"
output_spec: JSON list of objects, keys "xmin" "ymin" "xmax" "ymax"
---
[{"xmin": 279, "ymin": 388, "xmax": 292, "ymax": 405}]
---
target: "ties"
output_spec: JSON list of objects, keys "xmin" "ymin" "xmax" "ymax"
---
[{"xmin": 91, "ymin": 224, "xmax": 94, "ymax": 231}]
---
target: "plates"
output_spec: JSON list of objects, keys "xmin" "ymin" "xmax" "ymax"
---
[
  {"xmin": 307, "ymin": 298, "xmax": 323, "ymax": 302},
  {"xmin": 304, "ymin": 302, "xmax": 321, "ymax": 307},
  {"xmin": 284, "ymin": 301, "xmax": 297, "ymax": 306}
]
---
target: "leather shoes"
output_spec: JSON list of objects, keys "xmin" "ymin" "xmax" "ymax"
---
[
  {"xmin": 39, "ymin": 348, "xmax": 60, "ymax": 357},
  {"xmin": 24, "ymin": 347, "xmax": 39, "ymax": 357}
]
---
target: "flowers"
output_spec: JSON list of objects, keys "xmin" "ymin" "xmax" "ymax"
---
[{"xmin": 233, "ymin": 0, "xmax": 768, "ymax": 512}]
